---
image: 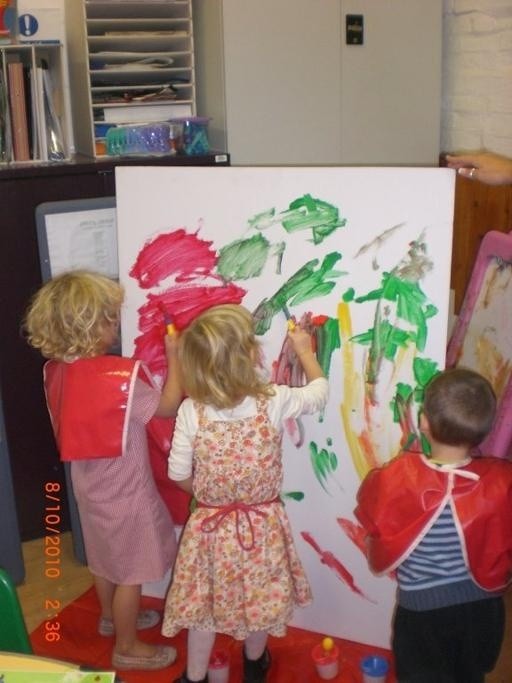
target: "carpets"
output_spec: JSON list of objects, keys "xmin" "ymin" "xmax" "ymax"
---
[{"xmin": 27, "ymin": 584, "xmax": 397, "ymax": 683}]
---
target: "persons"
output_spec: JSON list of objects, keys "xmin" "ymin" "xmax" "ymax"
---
[
  {"xmin": 353, "ymin": 368, "xmax": 512, "ymax": 683},
  {"xmin": 446, "ymin": 152, "xmax": 512, "ymax": 186},
  {"xmin": 20, "ymin": 271, "xmax": 183, "ymax": 670},
  {"xmin": 161, "ymin": 304, "xmax": 330, "ymax": 683}
]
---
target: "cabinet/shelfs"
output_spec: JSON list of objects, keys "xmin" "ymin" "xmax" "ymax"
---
[
  {"xmin": 193, "ymin": 2, "xmax": 446, "ymax": 167},
  {"xmin": 0, "ymin": 148, "xmax": 231, "ymax": 544},
  {"xmin": 65, "ymin": 0, "xmax": 197, "ymax": 158}
]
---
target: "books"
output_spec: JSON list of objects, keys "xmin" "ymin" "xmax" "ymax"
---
[
  {"xmin": 104, "ymin": 30, "xmax": 188, "ymax": 37},
  {"xmin": 103, "ymin": 105, "xmax": 192, "ymax": 121},
  {"xmin": 7, "ymin": 54, "xmax": 66, "ymax": 161}
]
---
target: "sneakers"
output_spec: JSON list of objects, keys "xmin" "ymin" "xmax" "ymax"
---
[
  {"xmin": 112, "ymin": 645, "xmax": 177, "ymax": 670},
  {"xmin": 99, "ymin": 609, "xmax": 161, "ymax": 636}
]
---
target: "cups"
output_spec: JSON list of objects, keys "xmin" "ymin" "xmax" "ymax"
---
[
  {"xmin": 360, "ymin": 655, "xmax": 388, "ymax": 683},
  {"xmin": 207, "ymin": 649, "xmax": 230, "ymax": 682},
  {"xmin": 312, "ymin": 645, "xmax": 340, "ymax": 679}
]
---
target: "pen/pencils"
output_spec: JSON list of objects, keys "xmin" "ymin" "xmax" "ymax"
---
[
  {"xmin": 288, "ymin": 319, "xmax": 295, "ymax": 332},
  {"xmin": 166, "ymin": 319, "xmax": 177, "ymax": 336}
]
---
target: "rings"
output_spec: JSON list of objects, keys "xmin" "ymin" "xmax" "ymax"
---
[{"xmin": 469, "ymin": 167, "xmax": 476, "ymax": 177}]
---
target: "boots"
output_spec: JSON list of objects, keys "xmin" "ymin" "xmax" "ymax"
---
[
  {"xmin": 173, "ymin": 670, "xmax": 208, "ymax": 683},
  {"xmin": 242, "ymin": 644, "xmax": 271, "ymax": 683}
]
